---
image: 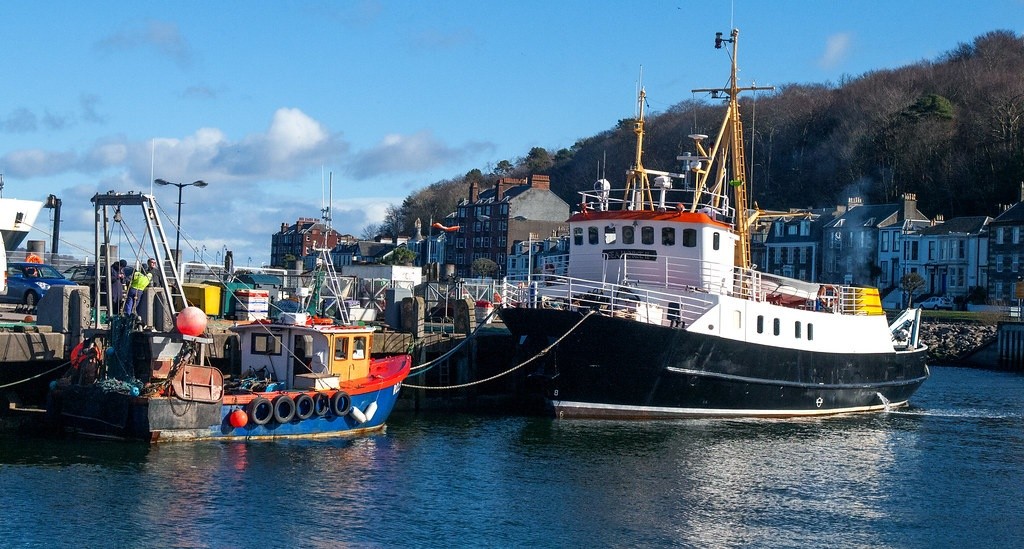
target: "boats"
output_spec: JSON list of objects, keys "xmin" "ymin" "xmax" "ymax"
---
[
  {"xmin": 41, "ymin": 170, "xmax": 417, "ymax": 443},
  {"xmin": 488, "ymin": 19, "xmax": 932, "ymax": 420}
]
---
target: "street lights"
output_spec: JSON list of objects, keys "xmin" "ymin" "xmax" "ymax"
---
[
  {"xmin": 154, "ymin": 179, "xmax": 210, "ymax": 311},
  {"xmin": 201, "ymin": 245, "xmax": 208, "ymax": 265},
  {"xmin": 216, "ymin": 251, "xmax": 221, "ymax": 264},
  {"xmin": 222, "ymin": 244, "xmax": 228, "ymax": 265},
  {"xmin": 193, "ymin": 247, "xmax": 199, "ymax": 262}
]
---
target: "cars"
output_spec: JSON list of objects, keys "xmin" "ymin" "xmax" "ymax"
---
[
  {"xmin": 0, "ymin": 262, "xmax": 80, "ymax": 307},
  {"xmin": 918, "ymin": 296, "xmax": 953, "ymax": 310},
  {"xmin": 59, "ymin": 264, "xmax": 126, "ymax": 309}
]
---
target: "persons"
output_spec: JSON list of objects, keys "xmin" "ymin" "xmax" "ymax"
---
[{"xmin": 109, "ymin": 257, "xmax": 162, "ymax": 316}]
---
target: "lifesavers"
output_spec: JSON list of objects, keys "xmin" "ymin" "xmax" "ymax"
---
[
  {"xmin": 292, "ymin": 394, "xmax": 314, "ymax": 420},
  {"xmin": 25, "ymin": 255, "xmax": 42, "ymax": 276},
  {"xmin": 272, "ymin": 394, "xmax": 295, "ymax": 423},
  {"xmin": 818, "ymin": 285, "xmax": 839, "ymax": 306},
  {"xmin": 247, "ymin": 397, "xmax": 273, "ymax": 425},
  {"xmin": 312, "ymin": 393, "xmax": 331, "ymax": 415},
  {"xmin": 331, "ymin": 392, "xmax": 351, "ymax": 416}
]
